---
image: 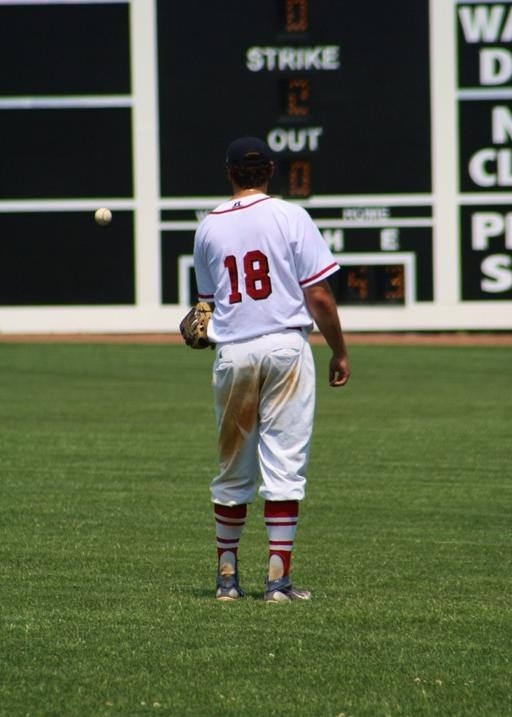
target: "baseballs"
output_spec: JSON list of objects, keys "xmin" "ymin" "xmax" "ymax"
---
[{"xmin": 94, "ymin": 209, "xmax": 110, "ymax": 221}]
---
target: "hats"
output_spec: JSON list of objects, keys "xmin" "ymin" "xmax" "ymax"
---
[{"xmin": 224, "ymin": 135, "xmax": 273, "ymax": 165}]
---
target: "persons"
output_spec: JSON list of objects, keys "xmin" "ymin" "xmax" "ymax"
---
[{"xmin": 180, "ymin": 136, "xmax": 351, "ymax": 606}]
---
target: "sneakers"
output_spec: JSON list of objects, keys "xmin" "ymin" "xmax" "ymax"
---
[
  {"xmin": 214, "ymin": 572, "xmax": 246, "ymax": 601},
  {"xmin": 264, "ymin": 575, "xmax": 312, "ymax": 604}
]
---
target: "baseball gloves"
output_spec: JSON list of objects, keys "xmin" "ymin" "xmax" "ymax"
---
[{"xmin": 179, "ymin": 303, "xmax": 215, "ymax": 349}]
